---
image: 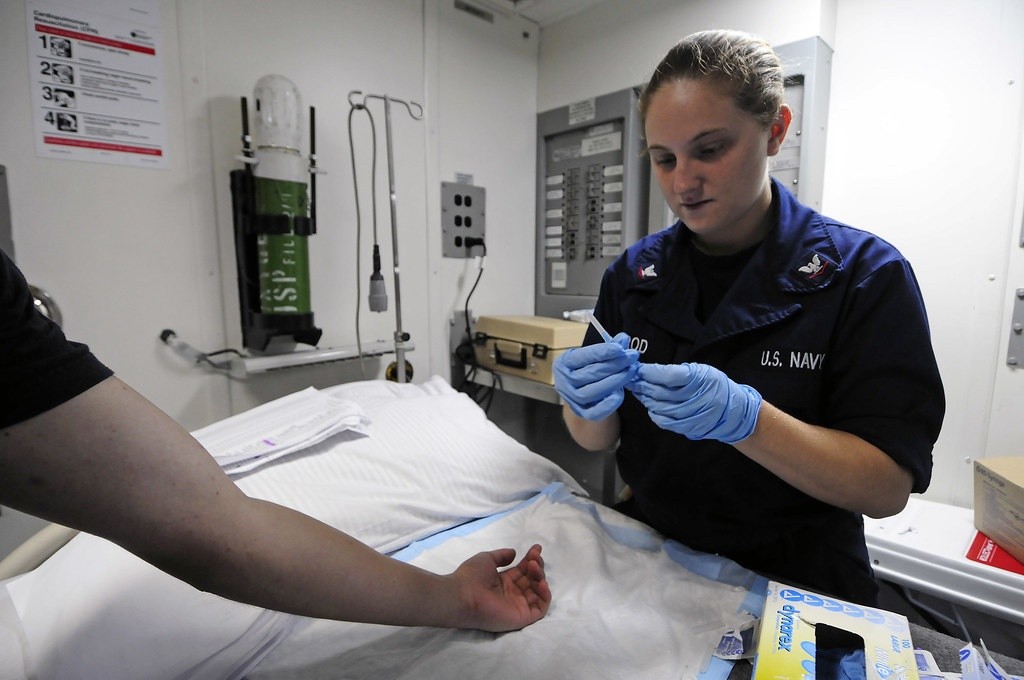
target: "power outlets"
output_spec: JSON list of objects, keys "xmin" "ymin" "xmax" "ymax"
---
[{"xmin": 441, "ymin": 181, "xmax": 487, "ymax": 259}]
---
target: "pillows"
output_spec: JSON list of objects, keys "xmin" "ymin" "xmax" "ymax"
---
[{"xmin": 0, "ymin": 374, "xmax": 591, "ymax": 680}]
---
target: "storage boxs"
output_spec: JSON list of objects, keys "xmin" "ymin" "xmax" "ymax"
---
[
  {"xmin": 473, "ymin": 315, "xmax": 585, "ymax": 386},
  {"xmin": 974, "ymin": 457, "xmax": 1024, "ymax": 564}
]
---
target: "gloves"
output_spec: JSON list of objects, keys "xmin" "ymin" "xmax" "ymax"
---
[
  {"xmin": 548, "ymin": 329, "xmax": 640, "ymax": 420},
  {"xmin": 625, "ymin": 356, "xmax": 764, "ymax": 445}
]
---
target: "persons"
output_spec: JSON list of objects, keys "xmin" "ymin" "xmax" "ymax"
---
[
  {"xmin": 0, "ymin": 251, "xmax": 554, "ymax": 633},
  {"xmin": 548, "ymin": 27, "xmax": 946, "ymax": 611}
]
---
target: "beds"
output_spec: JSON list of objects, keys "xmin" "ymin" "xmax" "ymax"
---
[{"xmin": 1, "ymin": 493, "xmax": 1024, "ymax": 680}]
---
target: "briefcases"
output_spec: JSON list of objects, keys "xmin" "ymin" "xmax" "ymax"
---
[{"xmin": 480, "ymin": 314, "xmax": 591, "ymax": 387}]
